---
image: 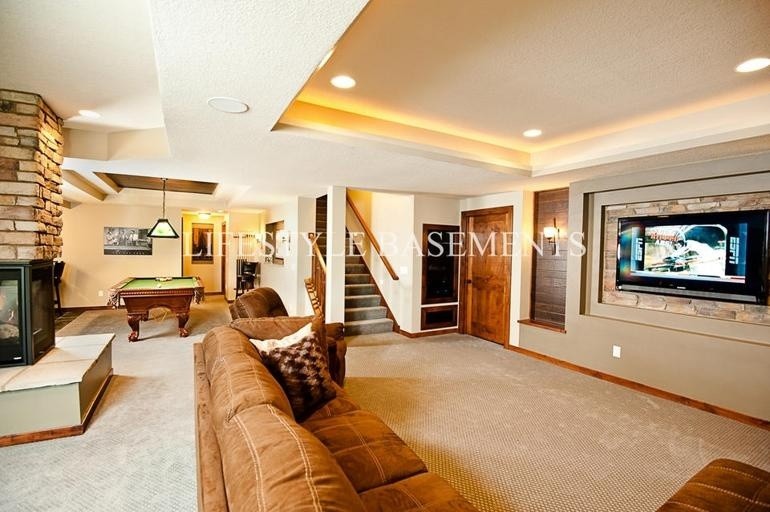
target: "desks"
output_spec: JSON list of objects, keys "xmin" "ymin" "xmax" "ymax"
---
[{"xmin": 107, "ymin": 274, "xmax": 206, "ymax": 343}]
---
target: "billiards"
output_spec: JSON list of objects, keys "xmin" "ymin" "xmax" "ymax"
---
[
  {"xmin": 155, "ymin": 277, "xmax": 172, "ymax": 282},
  {"xmin": 157, "ymin": 284, "xmax": 161, "ymax": 287}
]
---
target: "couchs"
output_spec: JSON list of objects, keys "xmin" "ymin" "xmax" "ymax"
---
[{"xmin": 192, "ymin": 322, "xmax": 479, "ymax": 512}]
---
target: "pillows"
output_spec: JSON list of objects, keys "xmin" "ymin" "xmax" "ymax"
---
[{"xmin": 232, "ymin": 313, "xmax": 340, "ymax": 424}]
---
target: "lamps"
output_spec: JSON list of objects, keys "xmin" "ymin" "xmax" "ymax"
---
[{"xmin": 147, "ymin": 176, "xmax": 179, "ymax": 239}]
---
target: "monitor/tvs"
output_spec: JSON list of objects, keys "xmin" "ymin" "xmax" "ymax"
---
[{"xmin": 616, "ymin": 209, "xmax": 770, "ymax": 309}]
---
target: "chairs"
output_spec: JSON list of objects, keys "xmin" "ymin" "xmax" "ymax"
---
[{"xmin": 228, "ymin": 287, "xmax": 347, "ymax": 387}]
